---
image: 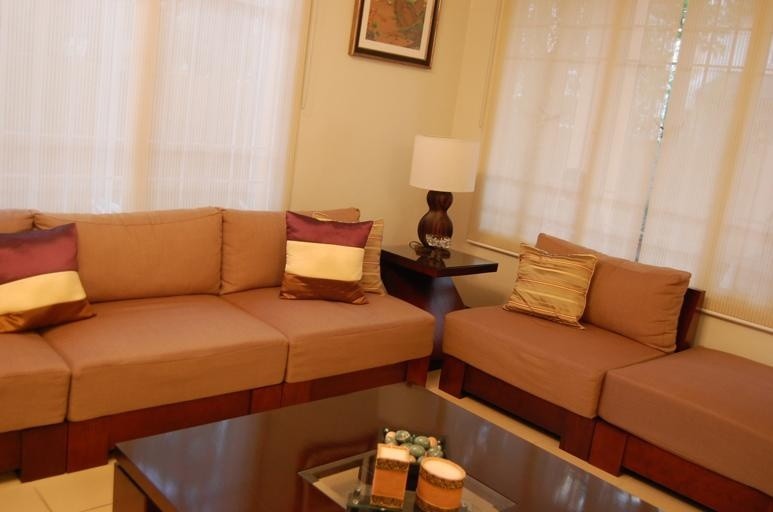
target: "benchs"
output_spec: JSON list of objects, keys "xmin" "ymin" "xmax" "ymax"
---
[{"xmin": 588, "ymin": 288, "xmax": 773, "ymax": 512}]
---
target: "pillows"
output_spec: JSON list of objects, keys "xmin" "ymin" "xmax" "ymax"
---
[
  {"xmin": 311, "ymin": 211, "xmax": 384, "ymax": 295},
  {"xmin": 502, "ymin": 242, "xmax": 600, "ymax": 330},
  {"xmin": 0, "ymin": 207, "xmax": 39, "ymax": 234},
  {"xmin": 222, "ymin": 209, "xmax": 360, "ymax": 293},
  {"xmin": 31, "ymin": 207, "xmax": 223, "ymax": 304},
  {"xmin": 0, "ymin": 223, "xmax": 97, "ymax": 334},
  {"xmin": 535, "ymin": 232, "xmax": 691, "ymax": 354},
  {"xmin": 280, "ymin": 211, "xmax": 373, "ymax": 304}
]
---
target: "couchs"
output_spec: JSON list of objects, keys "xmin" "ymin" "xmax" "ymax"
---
[
  {"xmin": 222, "ymin": 205, "xmax": 435, "ymax": 407},
  {"xmin": 33, "ymin": 207, "xmax": 289, "ymax": 484},
  {"xmin": 0, "ymin": 206, "xmax": 72, "ymax": 483},
  {"xmin": 442, "ymin": 235, "xmax": 704, "ymax": 458}
]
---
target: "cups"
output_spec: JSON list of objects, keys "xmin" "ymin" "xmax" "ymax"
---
[{"xmin": 415, "ymin": 455, "xmax": 465, "ymax": 511}]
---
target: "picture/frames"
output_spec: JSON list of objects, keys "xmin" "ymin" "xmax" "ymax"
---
[{"xmin": 348, "ymin": 0, "xmax": 442, "ymax": 70}]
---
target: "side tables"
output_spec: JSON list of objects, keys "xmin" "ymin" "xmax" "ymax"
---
[{"xmin": 381, "ymin": 245, "xmax": 498, "ymax": 374}]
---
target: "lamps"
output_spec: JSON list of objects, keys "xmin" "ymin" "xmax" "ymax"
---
[{"xmin": 409, "ymin": 135, "xmax": 481, "ymax": 249}]
